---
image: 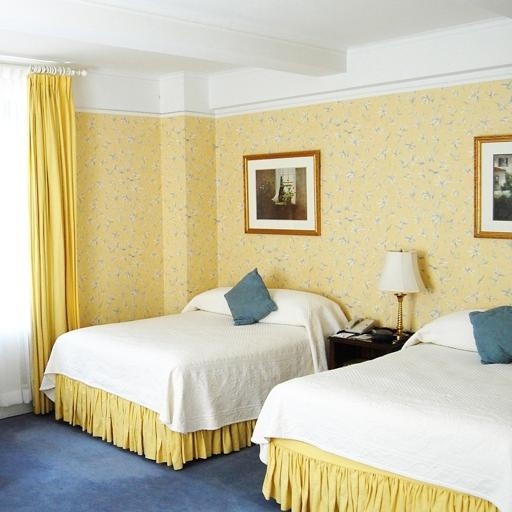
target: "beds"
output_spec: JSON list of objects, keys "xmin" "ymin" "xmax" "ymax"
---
[
  {"xmin": 52, "ymin": 287, "xmax": 346, "ymax": 468},
  {"xmin": 259, "ymin": 311, "xmax": 512, "ymax": 511}
]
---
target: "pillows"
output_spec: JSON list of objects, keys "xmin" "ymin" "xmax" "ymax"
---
[
  {"xmin": 223, "ymin": 267, "xmax": 278, "ymax": 325},
  {"xmin": 468, "ymin": 304, "xmax": 511, "ymax": 365}
]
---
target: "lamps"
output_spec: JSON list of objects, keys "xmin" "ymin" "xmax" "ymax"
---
[{"xmin": 377, "ymin": 249, "xmax": 428, "ymax": 339}]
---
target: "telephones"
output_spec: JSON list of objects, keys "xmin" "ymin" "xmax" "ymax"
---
[{"xmin": 344, "ymin": 317, "xmax": 376, "ymax": 334}]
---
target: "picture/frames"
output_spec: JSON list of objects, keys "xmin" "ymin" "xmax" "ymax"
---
[
  {"xmin": 241, "ymin": 149, "xmax": 324, "ymax": 238},
  {"xmin": 472, "ymin": 134, "xmax": 511, "ymax": 241}
]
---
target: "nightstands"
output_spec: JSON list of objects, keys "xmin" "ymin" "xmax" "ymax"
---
[{"xmin": 327, "ymin": 327, "xmax": 412, "ymax": 368}]
